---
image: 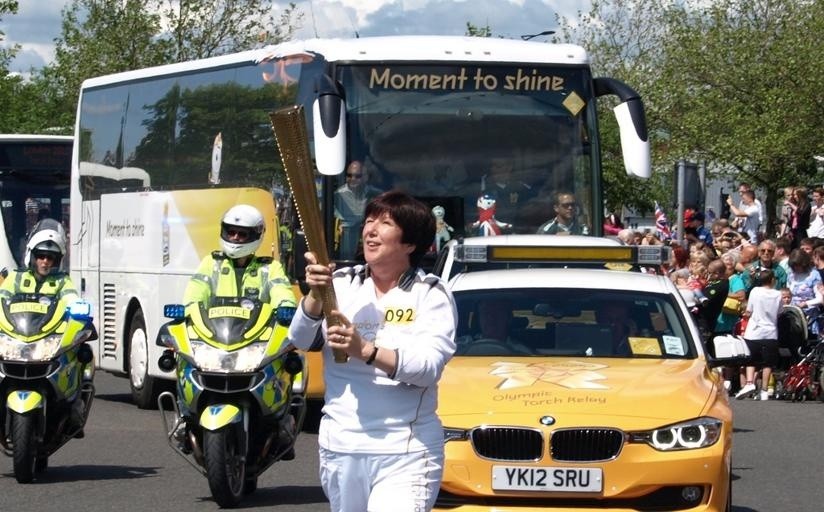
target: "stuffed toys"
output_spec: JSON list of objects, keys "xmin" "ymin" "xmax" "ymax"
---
[
  {"xmin": 468, "ymin": 191, "xmax": 515, "ymax": 236},
  {"xmin": 424, "ymin": 204, "xmax": 456, "ymax": 256}
]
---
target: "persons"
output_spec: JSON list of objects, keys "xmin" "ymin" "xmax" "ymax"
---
[
  {"xmin": 173, "ymin": 203, "xmax": 300, "ymax": 461},
  {"xmin": 479, "ymin": 150, "xmax": 537, "ymax": 219},
  {"xmin": 288, "ymin": 190, "xmax": 461, "ymax": 512},
  {"xmin": 590, "ymin": 300, "xmax": 638, "ymax": 355},
  {"xmin": 453, "ymin": 298, "xmax": 535, "ymax": 356},
  {"xmin": 1, "ymin": 218, "xmax": 96, "ymax": 442},
  {"xmin": 333, "ymin": 152, "xmax": 382, "ymax": 249},
  {"xmin": 537, "ymin": 189, "xmax": 592, "ymax": 237},
  {"xmin": 604, "ymin": 184, "xmax": 823, "ymax": 403}
]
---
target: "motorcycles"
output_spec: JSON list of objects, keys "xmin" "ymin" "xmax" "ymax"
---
[
  {"xmin": 154, "ymin": 303, "xmax": 314, "ymax": 512},
  {"xmin": 0, "ymin": 285, "xmax": 98, "ymax": 490}
]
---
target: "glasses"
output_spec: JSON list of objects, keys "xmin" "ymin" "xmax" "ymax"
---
[
  {"xmin": 34, "ymin": 253, "xmax": 57, "ymax": 262},
  {"xmin": 224, "ymin": 227, "xmax": 252, "ymax": 241},
  {"xmin": 344, "ymin": 171, "xmax": 366, "ymax": 179},
  {"xmin": 557, "ymin": 202, "xmax": 577, "ymax": 209},
  {"xmin": 760, "ymin": 249, "xmax": 776, "ymax": 254}
]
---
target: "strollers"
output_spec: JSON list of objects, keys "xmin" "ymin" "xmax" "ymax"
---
[
  {"xmin": 773, "ymin": 337, "xmax": 824, "ymax": 402},
  {"xmin": 775, "ymin": 303, "xmax": 819, "ymax": 379}
]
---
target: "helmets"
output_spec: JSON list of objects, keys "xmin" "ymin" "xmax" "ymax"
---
[
  {"xmin": 24, "ymin": 217, "xmax": 66, "ymax": 273},
  {"xmin": 219, "ymin": 204, "xmax": 266, "ymax": 259}
]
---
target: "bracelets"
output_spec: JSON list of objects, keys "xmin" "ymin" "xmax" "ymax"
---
[{"xmin": 364, "ymin": 347, "xmax": 378, "ymax": 365}]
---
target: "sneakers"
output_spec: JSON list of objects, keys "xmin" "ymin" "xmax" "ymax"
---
[
  {"xmin": 699, "ymin": 296, "xmax": 710, "ymax": 307},
  {"xmin": 735, "ymin": 384, "xmax": 769, "ymax": 401}
]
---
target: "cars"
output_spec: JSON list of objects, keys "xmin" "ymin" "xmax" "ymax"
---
[
  {"xmin": 407, "ymin": 229, "xmax": 740, "ymax": 512},
  {"xmin": 424, "ymin": 231, "xmax": 640, "ymax": 289}
]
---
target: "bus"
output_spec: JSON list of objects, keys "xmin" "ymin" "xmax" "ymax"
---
[
  {"xmin": 63, "ymin": 26, "xmax": 656, "ymax": 436},
  {"xmin": 0, "ymin": 131, "xmax": 73, "ymax": 286}
]
